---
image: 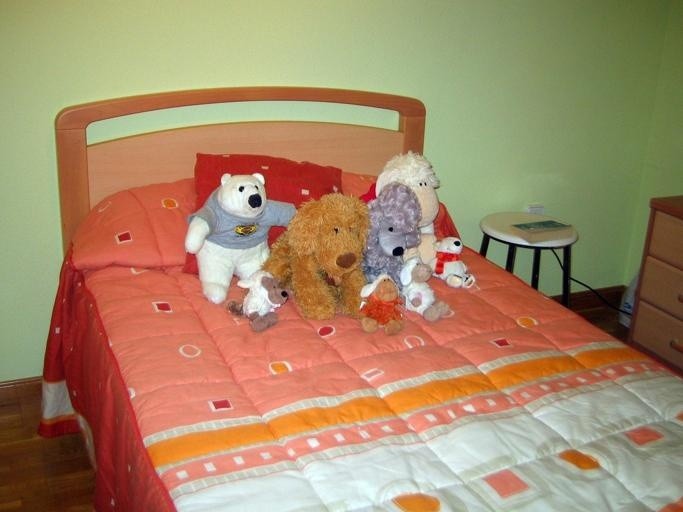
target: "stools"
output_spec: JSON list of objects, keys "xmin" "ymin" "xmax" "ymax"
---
[{"xmin": 479, "ymin": 212, "xmax": 578, "ymax": 308}]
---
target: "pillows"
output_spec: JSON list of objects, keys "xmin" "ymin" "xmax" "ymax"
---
[{"xmin": 186, "ymin": 151, "xmax": 342, "ymax": 277}]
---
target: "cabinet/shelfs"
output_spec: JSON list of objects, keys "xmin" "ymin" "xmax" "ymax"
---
[{"xmin": 628, "ymin": 194, "xmax": 683, "ymax": 366}]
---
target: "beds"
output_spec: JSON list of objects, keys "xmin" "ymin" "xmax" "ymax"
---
[{"xmin": 39, "ymin": 86, "xmax": 683, "ymax": 512}]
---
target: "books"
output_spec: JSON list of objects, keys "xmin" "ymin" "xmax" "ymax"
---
[{"xmin": 510, "ymin": 220, "xmax": 573, "ymax": 244}]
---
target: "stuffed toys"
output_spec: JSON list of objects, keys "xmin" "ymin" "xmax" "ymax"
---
[
  {"xmin": 184, "ymin": 172, "xmax": 298, "ymax": 305},
  {"xmin": 428, "ymin": 236, "xmax": 475, "ymax": 289},
  {"xmin": 360, "ymin": 274, "xmax": 405, "ymax": 335},
  {"xmin": 228, "ymin": 269, "xmax": 289, "ymax": 333},
  {"xmin": 375, "ymin": 151, "xmax": 440, "ymax": 274},
  {"xmin": 361, "ymin": 182, "xmax": 423, "ymax": 290},
  {"xmin": 397, "ymin": 257, "xmax": 449, "ymax": 323},
  {"xmin": 262, "ymin": 191, "xmax": 371, "ymax": 320}
]
